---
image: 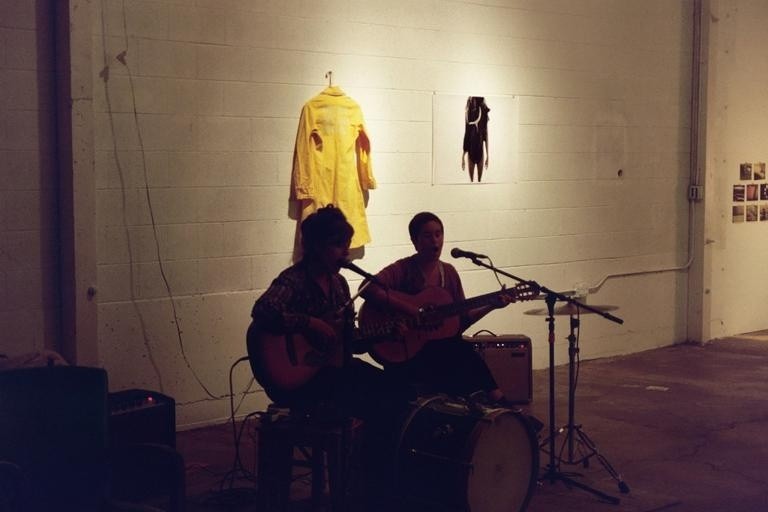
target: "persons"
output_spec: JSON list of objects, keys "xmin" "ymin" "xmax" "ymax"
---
[
  {"xmin": 251, "ymin": 205, "xmax": 402, "ymax": 511},
  {"xmin": 359, "ymin": 212, "xmax": 545, "ymax": 437},
  {"xmin": 461, "ymin": 96, "xmax": 491, "ymax": 182}
]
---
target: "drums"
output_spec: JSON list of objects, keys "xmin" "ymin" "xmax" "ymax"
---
[{"xmin": 382, "ymin": 388, "xmax": 539, "ymax": 512}]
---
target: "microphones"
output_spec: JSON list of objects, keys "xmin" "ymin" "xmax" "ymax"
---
[
  {"xmin": 340, "ymin": 255, "xmax": 392, "ymax": 296},
  {"xmin": 448, "ymin": 247, "xmax": 490, "ymax": 267}
]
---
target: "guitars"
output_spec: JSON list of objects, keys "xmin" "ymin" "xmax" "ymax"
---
[
  {"xmin": 358, "ymin": 280, "xmax": 540, "ymax": 366},
  {"xmin": 249, "ymin": 295, "xmax": 442, "ymax": 398}
]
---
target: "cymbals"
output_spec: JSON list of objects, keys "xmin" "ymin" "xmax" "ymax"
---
[
  {"xmin": 524, "ymin": 302, "xmax": 620, "ymax": 315},
  {"xmin": 526, "ymin": 291, "xmax": 576, "ymax": 300}
]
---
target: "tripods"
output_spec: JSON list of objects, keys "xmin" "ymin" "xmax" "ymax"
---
[{"xmin": 537, "ymin": 318, "xmax": 632, "ymax": 506}]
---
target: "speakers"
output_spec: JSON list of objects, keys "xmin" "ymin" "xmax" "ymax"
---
[{"xmin": 456, "ymin": 333, "xmax": 534, "ymax": 407}]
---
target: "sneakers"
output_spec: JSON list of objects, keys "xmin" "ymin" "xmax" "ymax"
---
[{"xmin": 509, "ymin": 407, "xmax": 545, "ymax": 434}]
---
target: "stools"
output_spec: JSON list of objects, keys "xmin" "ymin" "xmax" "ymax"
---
[{"xmin": 255, "ymin": 401, "xmax": 365, "ymax": 506}]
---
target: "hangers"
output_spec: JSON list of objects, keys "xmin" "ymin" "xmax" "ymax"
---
[{"xmin": 308, "ymin": 71, "xmax": 360, "ymax": 107}]
